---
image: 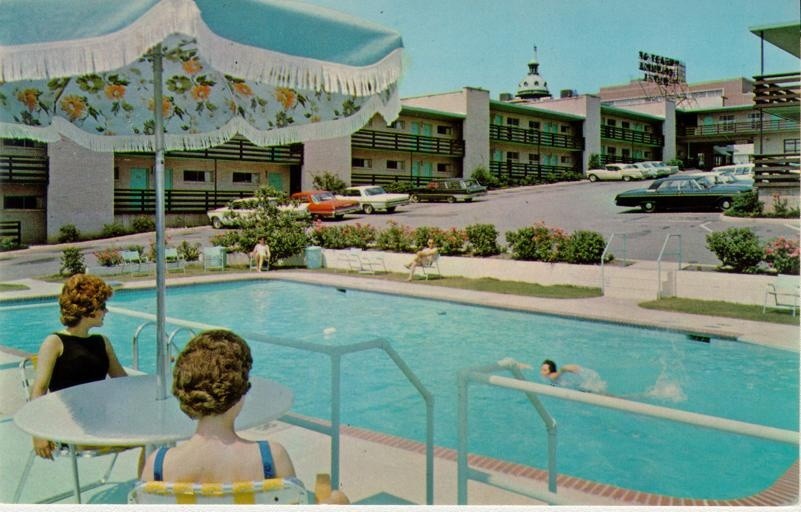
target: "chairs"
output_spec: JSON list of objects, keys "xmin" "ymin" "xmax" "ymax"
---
[
  {"xmin": 306, "ymin": 194, "xmax": 326, "ymax": 205},
  {"xmin": 118, "ymin": 246, "xmax": 275, "ymax": 278},
  {"xmin": 763, "ymin": 273, "xmax": 800, "ymax": 317},
  {"xmin": 335, "ymin": 244, "xmax": 443, "ymax": 281},
  {"xmin": 127, "ymin": 477, "xmax": 308, "ymax": 503},
  {"xmin": 664, "ymin": 179, "xmax": 714, "ymax": 195},
  {"xmin": 13, "ymin": 355, "xmax": 126, "ymax": 504}
]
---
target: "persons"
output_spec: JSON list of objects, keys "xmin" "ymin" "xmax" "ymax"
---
[
  {"xmin": 520, "ymin": 357, "xmax": 665, "ymax": 399},
  {"xmin": 27, "ymin": 272, "xmax": 147, "ymax": 461},
  {"xmin": 246, "ymin": 235, "xmax": 271, "ymax": 273},
  {"xmin": 404, "ymin": 237, "xmax": 440, "ymax": 281},
  {"xmin": 139, "ymin": 329, "xmax": 352, "ymax": 503}
]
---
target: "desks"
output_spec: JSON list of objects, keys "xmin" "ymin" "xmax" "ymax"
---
[{"xmin": 13, "ymin": 370, "xmax": 294, "ymax": 451}]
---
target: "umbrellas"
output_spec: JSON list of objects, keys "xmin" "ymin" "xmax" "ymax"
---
[{"xmin": 1, "ymin": 0, "xmax": 409, "ymax": 396}]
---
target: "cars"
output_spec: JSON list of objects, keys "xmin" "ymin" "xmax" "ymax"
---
[
  {"xmin": 206, "ymin": 179, "xmax": 488, "ymax": 231},
  {"xmin": 587, "ymin": 160, "xmax": 800, "ymax": 214}
]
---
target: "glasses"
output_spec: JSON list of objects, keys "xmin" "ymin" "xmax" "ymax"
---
[{"xmin": 95, "ymin": 302, "xmax": 104, "ymax": 310}]
